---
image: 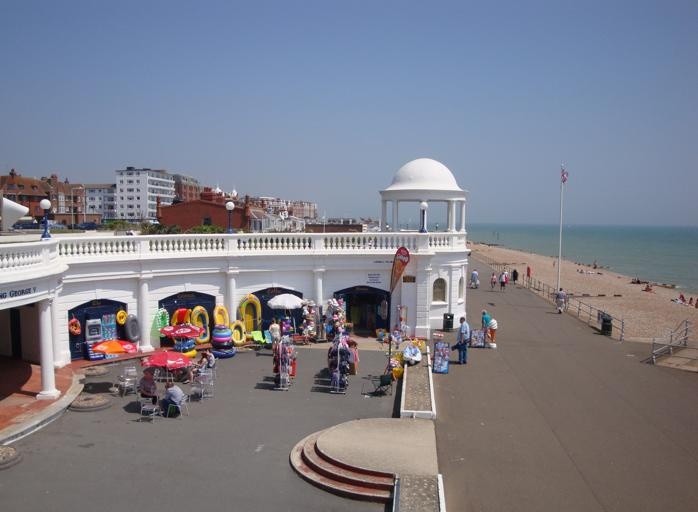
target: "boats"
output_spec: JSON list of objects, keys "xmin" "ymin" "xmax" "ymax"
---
[{"xmin": 123, "ymin": 293, "xmax": 263, "ymax": 347}]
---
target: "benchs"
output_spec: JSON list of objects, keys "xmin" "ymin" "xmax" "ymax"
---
[
  {"xmin": 163, "ymin": 343, "xmax": 213, "ymax": 352},
  {"xmin": 371, "ymin": 375, "xmax": 392, "ymax": 397}
]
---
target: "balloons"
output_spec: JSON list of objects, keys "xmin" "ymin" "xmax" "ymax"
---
[{"xmin": 282, "ymin": 319, "xmax": 294, "ymax": 333}]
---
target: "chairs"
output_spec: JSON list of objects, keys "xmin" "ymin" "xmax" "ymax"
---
[
  {"xmin": 264, "ymin": 330, "xmax": 272, "ymax": 343},
  {"xmin": 251, "ymin": 331, "xmax": 266, "ymax": 351},
  {"xmin": 112, "ymin": 357, "xmax": 218, "ymax": 425}
]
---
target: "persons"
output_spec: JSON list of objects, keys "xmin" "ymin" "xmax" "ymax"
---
[
  {"xmin": 679, "ymin": 294, "xmax": 698, "ymax": 308},
  {"xmin": 139, "ymin": 367, "xmax": 159, "ymax": 405},
  {"xmin": 645, "ymin": 284, "xmax": 651, "ymax": 291},
  {"xmin": 455, "ymin": 317, "xmax": 471, "ymax": 364},
  {"xmin": 554, "ymin": 287, "xmax": 566, "ymax": 313},
  {"xmin": 204, "ymin": 348, "xmax": 217, "ymax": 368},
  {"xmin": 181, "ymin": 351, "xmax": 209, "ymax": 384},
  {"xmin": 162, "ymin": 381, "xmax": 185, "ymax": 417},
  {"xmin": 481, "ymin": 309, "xmax": 498, "ymax": 343},
  {"xmin": 471, "ymin": 269, "xmax": 518, "ymax": 292},
  {"xmin": 268, "ymin": 317, "xmax": 282, "ymax": 356}
]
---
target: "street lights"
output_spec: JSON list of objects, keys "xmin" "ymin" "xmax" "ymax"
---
[
  {"xmin": 222, "ymin": 201, "xmax": 236, "ymax": 233},
  {"xmin": 38, "ymin": 198, "xmax": 52, "ymax": 238},
  {"xmin": 418, "ymin": 199, "xmax": 429, "ymax": 233}
]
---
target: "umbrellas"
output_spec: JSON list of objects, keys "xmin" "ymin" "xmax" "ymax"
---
[
  {"xmin": 161, "ymin": 322, "xmax": 202, "ymax": 348},
  {"xmin": 266, "ymin": 293, "xmax": 304, "ymax": 316},
  {"xmin": 140, "ymin": 349, "xmax": 193, "ymax": 380},
  {"xmin": 90, "ymin": 339, "xmax": 138, "ymax": 387}
]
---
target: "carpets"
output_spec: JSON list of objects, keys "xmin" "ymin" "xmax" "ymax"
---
[{"xmin": 671, "ymin": 298, "xmax": 698, "ymax": 310}]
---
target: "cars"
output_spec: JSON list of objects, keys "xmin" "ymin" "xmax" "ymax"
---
[
  {"xmin": 16, "ymin": 219, "xmax": 66, "ymax": 231},
  {"xmin": 68, "ymin": 223, "xmax": 81, "ymax": 230},
  {"xmin": 80, "ymin": 222, "xmax": 97, "ymax": 230}
]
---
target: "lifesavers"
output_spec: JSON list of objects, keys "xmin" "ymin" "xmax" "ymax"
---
[
  {"xmin": 174, "ymin": 336, "xmax": 197, "ymax": 360},
  {"xmin": 69, "ymin": 318, "xmax": 82, "ymax": 335},
  {"xmin": 208, "ymin": 325, "xmax": 236, "ymax": 358},
  {"xmin": 117, "ymin": 312, "xmax": 128, "ymax": 327}
]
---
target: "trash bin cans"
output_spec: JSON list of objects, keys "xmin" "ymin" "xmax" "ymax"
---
[
  {"xmin": 443, "ymin": 313, "xmax": 454, "ymax": 332},
  {"xmin": 602, "ymin": 316, "xmax": 613, "ymax": 336}
]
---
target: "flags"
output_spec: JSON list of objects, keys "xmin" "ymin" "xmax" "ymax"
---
[{"xmin": 562, "ymin": 171, "xmax": 568, "ymax": 183}]
